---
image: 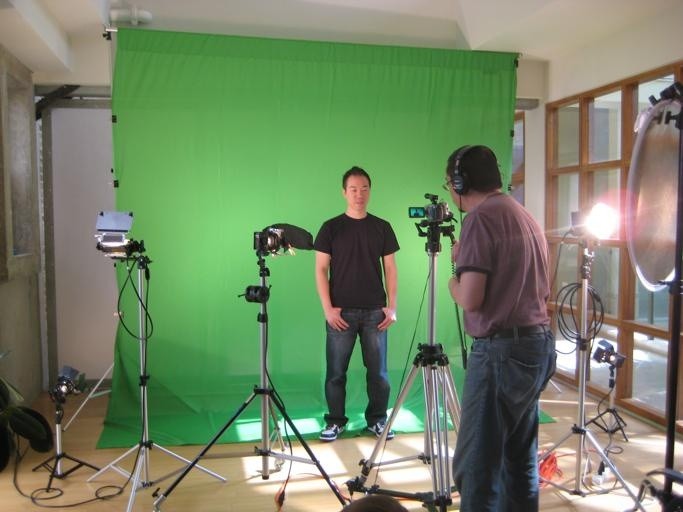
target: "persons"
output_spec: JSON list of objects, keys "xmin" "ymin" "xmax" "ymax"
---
[
  {"xmin": 313, "ymin": 166, "xmax": 400, "ymax": 442},
  {"xmin": 444, "ymin": 144, "xmax": 558, "ymax": 512}
]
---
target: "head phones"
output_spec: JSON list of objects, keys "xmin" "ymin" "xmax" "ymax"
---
[{"xmin": 451, "ymin": 144, "xmax": 500, "ymax": 195}]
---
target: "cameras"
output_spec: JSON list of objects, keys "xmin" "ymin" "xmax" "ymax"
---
[
  {"xmin": 407, "ymin": 192, "xmax": 459, "ymax": 226},
  {"xmin": 249, "ymin": 225, "xmax": 291, "ymax": 255}
]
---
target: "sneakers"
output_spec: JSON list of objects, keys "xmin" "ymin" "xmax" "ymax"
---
[
  {"xmin": 320, "ymin": 422, "xmax": 344, "ymax": 441},
  {"xmin": 365, "ymin": 422, "xmax": 394, "ymax": 440}
]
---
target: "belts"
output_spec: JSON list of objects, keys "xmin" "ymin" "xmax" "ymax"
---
[{"xmin": 473, "ymin": 325, "xmax": 551, "ymax": 338}]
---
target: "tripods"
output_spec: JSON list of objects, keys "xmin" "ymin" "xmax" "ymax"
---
[
  {"xmin": 88, "ymin": 257, "xmax": 226, "ymax": 511},
  {"xmin": 537, "ymin": 248, "xmax": 648, "ymax": 512},
  {"xmin": 585, "ymin": 367, "xmax": 631, "ymax": 442},
  {"xmin": 345, "ymin": 223, "xmax": 462, "ymax": 512},
  {"xmin": 154, "ymin": 259, "xmax": 347, "ymax": 511},
  {"xmin": 31, "ymin": 395, "xmax": 101, "ymax": 494}
]
